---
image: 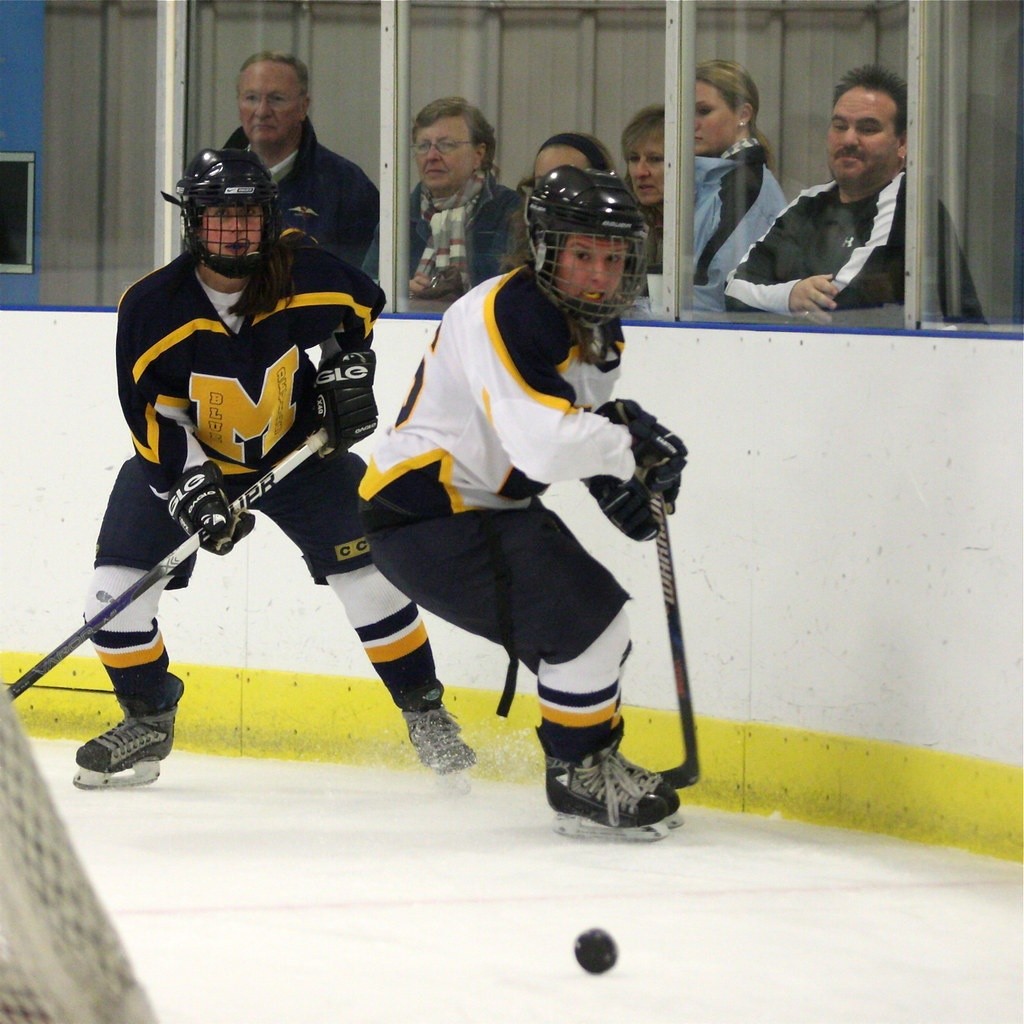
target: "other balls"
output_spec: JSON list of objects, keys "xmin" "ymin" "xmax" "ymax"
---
[{"xmin": 575, "ymin": 928, "xmax": 619, "ymax": 974}]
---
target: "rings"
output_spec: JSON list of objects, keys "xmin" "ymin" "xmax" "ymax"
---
[{"xmin": 804, "ymin": 311, "xmax": 808, "ymax": 317}]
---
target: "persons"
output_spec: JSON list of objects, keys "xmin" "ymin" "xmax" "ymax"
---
[
  {"xmin": 723, "ymin": 64, "xmax": 989, "ymax": 331},
  {"xmin": 72, "ymin": 148, "xmax": 479, "ymax": 792},
  {"xmin": 359, "ymin": 96, "xmax": 534, "ymax": 300},
  {"xmin": 687, "ymin": 57, "xmax": 788, "ymax": 311},
  {"xmin": 218, "ymin": 50, "xmax": 380, "ymax": 281},
  {"xmin": 499, "ymin": 131, "xmax": 614, "ymax": 273},
  {"xmin": 357, "ymin": 165, "xmax": 688, "ymax": 841},
  {"xmin": 619, "ymin": 105, "xmax": 665, "ymax": 297}
]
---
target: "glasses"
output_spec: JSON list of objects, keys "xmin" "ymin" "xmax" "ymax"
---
[{"xmin": 409, "ymin": 137, "xmax": 471, "ymax": 155}]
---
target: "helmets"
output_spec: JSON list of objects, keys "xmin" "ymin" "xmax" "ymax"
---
[
  {"xmin": 524, "ymin": 165, "xmax": 650, "ymax": 329},
  {"xmin": 161, "ymin": 148, "xmax": 282, "ymax": 277}
]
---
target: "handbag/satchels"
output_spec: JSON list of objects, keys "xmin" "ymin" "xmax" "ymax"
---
[{"xmin": 411, "ymin": 266, "xmax": 463, "ymax": 302}]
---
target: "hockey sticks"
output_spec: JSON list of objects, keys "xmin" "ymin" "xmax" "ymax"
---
[
  {"xmin": 8, "ymin": 426, "xmax": 331, "ymax": 703},
  {"xmin": 652, "ymin": 460, "xmax": 701, "ymax": 792}
]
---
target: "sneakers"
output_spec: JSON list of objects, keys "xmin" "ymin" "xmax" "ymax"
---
[
  {"xmin": 610, "ymin": 719, "xmax": 685, "ymax": 829},
  {"xmin": 535, "ymin": 724, "xmax": 670, "ymax": 841},
  {"xmin": 73, "ymin": 673, "xmax": 185, "ymax": 789},
  {"xmin": 401, "ymin": 682, "xmax": 478, "ymax": 797}
]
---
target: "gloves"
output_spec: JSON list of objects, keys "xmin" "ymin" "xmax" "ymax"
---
[
  {"xmin": 316, "ymin": 349, "xmax": 378, "ymax": 461},
  {"xmin": 166, "ymin": 460, "xmax": 255, "ymax": 556},
  {"xmin": 629, "ymin": 417, "xmax": 687, "ymax": 514},
  {"xmin": 592, "ymin": 474, "xmax": 661, "ymax": 542}
]
